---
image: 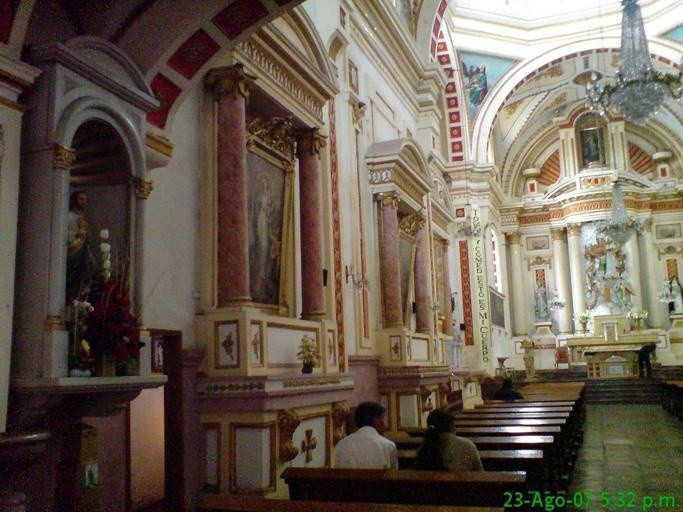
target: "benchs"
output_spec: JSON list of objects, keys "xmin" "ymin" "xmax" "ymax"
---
[{"xmin": 193, "ymin": 380, "xmax": 587, "ymax": 512}]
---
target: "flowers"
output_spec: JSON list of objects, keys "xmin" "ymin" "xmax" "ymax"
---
[
  {"xmin": 572, "ymin": 312, "xmax": 592, "ymax": 334},
  {"xmin": 626, "ymin": 307, "xmax": 649, "ymax": 330}
]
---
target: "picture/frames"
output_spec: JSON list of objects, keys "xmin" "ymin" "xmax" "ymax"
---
[
  {"xmin": 245, "ymin": 130, "xmax": 297, "ymax": 318},
  {"xmin": 399, "ymin": 228, "xmax": 418, "ymax": 329}
]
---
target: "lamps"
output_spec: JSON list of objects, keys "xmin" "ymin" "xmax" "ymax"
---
[{"xmin": 585, "ymin": 1, "xmax": 683, "ymax": 246}]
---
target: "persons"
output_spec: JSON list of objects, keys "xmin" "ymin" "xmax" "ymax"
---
[
  {"xmin": 252, "ymin": 175, "xmax": 277, "ymax": 291},
  {"xmin": 493, "ymin": 378, "xmax": 524, "ymax": 400},
  {"xmin": 415, "ymin": 408, "xmax": 485, "ymax": 471},
  {"xmin": 66, "ymin": 191, "xmax": 93, "ymax": 307},
  {"xmin": 331, "ymin": 402, "xmax": 399, "ymax": 470},
  {"xmin": 466, "ymin": 64, "xmax": 487, "ymax": 107},
  {"xmin": 638, "ymin": 343, "xmax": 656, "ymax": 377}
]
